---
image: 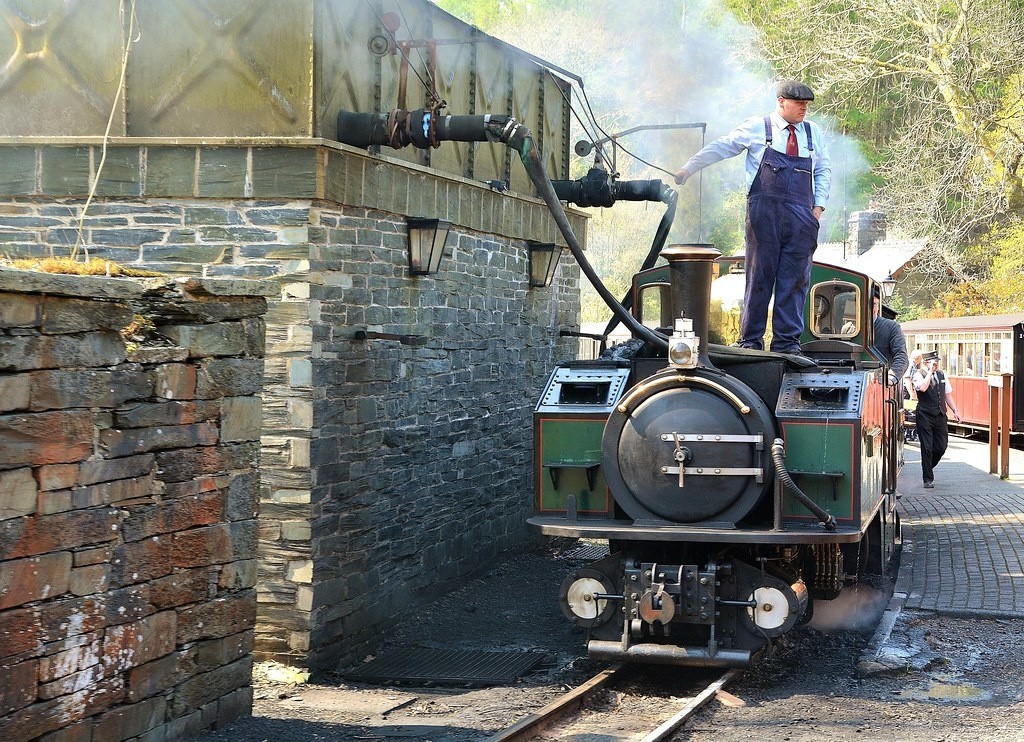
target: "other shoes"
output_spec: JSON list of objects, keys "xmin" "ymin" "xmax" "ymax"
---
[
  {"xmin": 782, "ymin": 349, "xmax": 816, "ymax": 364},
  {"xmin": 924, "ymin": 482, "xmax": 934, "ymax": 488}
]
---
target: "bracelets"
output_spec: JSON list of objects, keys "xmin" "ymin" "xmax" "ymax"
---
[{"xmin": 953, "ymin": 409, "xmax": 958, "ymax": 413}]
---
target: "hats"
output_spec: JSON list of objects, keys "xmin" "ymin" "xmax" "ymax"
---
[
  {"xmin": 922, "ymin": 350, "xmax": 941, "ymax": 363},
  {"xmin": 874, "ymin": 289, "xmax": 880, "ymax": 299},
  {"xmin": 777, "ymin": 80, "xmax": 814, "ymax": 100}
]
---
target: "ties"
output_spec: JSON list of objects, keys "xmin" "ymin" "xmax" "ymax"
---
[
  {"xmin": 932, "ymin": 372, "xmax": 939, "ymax": 383},
  {"xmin": 786, "ymin": 125, "xmax": 799, "ymax": 157}
]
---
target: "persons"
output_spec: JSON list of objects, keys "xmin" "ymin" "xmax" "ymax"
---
[
  {"xmin": 903, "ymin": 350, "xmax": 963, "ymax": 488},
  {"xmin": 840, "ymin": 290, "xmax": 909, "ymax": 387},
  {"xmin": 675, "ymin": 81, "xmax": 832, "ymax": 362}
]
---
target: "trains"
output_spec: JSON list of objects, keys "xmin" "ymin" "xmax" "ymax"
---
[
  {"xmin": 899, "ymin": 312, "xmax": 1024, "ymax": 440},
  {"xmin": 525, "ymin": 242, "xmax": 906, "ymax": 667}
]
